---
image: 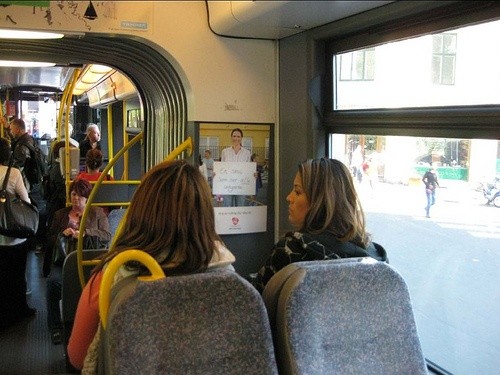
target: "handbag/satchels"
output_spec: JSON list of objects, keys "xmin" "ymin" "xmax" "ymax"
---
[
  {"xmin": 106, "ymin": 174, "xmax": 120, "ymax": 214},
  {"xmin": 51, "ymin": 232, "xmax": 106, "ymax": 265},
  {"xmin": 0, "ymin": 166, "xmax": 39, "ymax": 237}
]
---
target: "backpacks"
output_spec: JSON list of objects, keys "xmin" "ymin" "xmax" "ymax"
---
[
  {"xmin": 39, "ymin": 164, "xmax": 60, "ymax": 200},
  {"xmin": 17, "ymin": 137, "xmax": 45, "ymax": 190}
]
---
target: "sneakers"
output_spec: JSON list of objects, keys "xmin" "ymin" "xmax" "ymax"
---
[
  {"xmin": 51, "ymin": 328, "xmax": 63, "ymax": 344},
  {"xmin": 34, "ymin": 245, "xmax": 42, "ymax": 254}
]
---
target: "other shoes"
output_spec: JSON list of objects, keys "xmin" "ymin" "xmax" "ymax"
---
[{"xmin": 24, "ymin": 307, "xmax": 36, "ymax": 317}]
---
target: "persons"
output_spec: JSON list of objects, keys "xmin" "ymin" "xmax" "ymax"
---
[
  {"xmin": 351, "ymin": 146, "xmax": 378, "ymax": 188},
  {"xmin": 252, "ymin": 158, "xmax": 389, "ymax": 293},
  {"xmin": 200, "ymin": 129, "xmax": 268, "ymax": 206},
  {"xmin": 422, "ymin": 168, "xmax": 446, "ymax": 218},
  {"xmin": 1, "ymin": 117, "xmax": 111, "ymax": 345},
  {"xmin": 65, "ymin": 160, "xmax": 236, "ymax": 368}
]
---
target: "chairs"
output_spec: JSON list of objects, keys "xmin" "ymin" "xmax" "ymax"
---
[
  {"xmin": 280, "ymin": 261, "xmax": 427, "ymax": 375},
  {"xmin": 59, "ymin": 250, "xmax": 109, "ymax": 375},
  {"xmin": 105, "ymin": 270, "xmax": 278, "ymax": 375},
  {"xmin": 262, "ymin": 256, "xmax": 377, "ymax": 362}
]
